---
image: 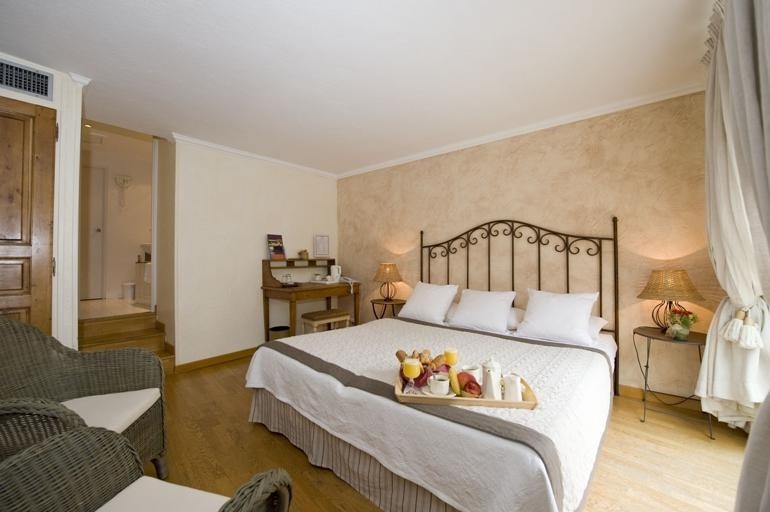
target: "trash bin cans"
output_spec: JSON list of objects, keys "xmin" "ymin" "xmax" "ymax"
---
[
  {"xmin": 268, "ymin": 326, "xmax": 291, "ymax": 340},
  {"xmin": 121, "ymin": 282, "xmax": 135, "ymax": 301}
]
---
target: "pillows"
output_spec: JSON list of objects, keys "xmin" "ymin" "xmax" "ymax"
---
[
  {"xmin": 589, "ymin": 316, "xmax": 608, "ymax": 346},
  {"xmin": 507, "ymin": 308, "xmax": 526, "ymax": 329},
  {"xmin": 444, "ymin": 303, "xmax": 458, "ymax": 321},
  {"xmin": 449, "ymin": 289, "xmax": 516, "ymax": 336},
  {"xmin": 398, "ymin": 281, "xmax": 460, "ymax": 327},
  {"xmin": 514, "ymin": 288, "xmax": 599, "ymax": 346}
]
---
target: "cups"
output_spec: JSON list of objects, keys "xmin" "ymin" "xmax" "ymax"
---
[
  {"xmin": 403, "ymin": 358, "xmax": 421, "ymax": 394},
  {"xmin": 427, "ymin": 374, "xmax": 450, "ymax": 394},
  {"xmin": 463, "ymin": 365, "xmax": 480, "ymax": 382},
  {"xmin": 444, "ymin": 345, "xmax": 457, "ymax": 366}
]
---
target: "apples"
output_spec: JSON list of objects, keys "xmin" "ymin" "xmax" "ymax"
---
[
  {"xmin": 464, "ymin": 381, "xmax": 481, "ymax": 396},
  {"xmin": 457, "ymin": 372, "xmax": 475, "ymax": 389}
]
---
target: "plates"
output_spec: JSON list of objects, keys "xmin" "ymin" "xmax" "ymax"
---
[
  {"xmin": 396, "ymin": 373, "xmax": 538, "ymax": 410},
  {"xmin": 421, "ymin": 386, "xmax": 456, "ymax": 397}
]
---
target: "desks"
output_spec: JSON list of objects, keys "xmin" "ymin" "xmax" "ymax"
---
[
  {"xmin": 633, "ymin": 326, "xmax": 716, "ymax": 440},
  {"xmin": 261, "ymin": 258, "xmax": 362, "ymax": 342},
  {"xmin": 370, "ymin": 299, "xmax": 407, "ymax": 319}
]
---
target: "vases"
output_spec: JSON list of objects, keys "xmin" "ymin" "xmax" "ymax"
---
[{"xmin": 665, "ymin": 324, "xmax": 689, "ymax": 341}]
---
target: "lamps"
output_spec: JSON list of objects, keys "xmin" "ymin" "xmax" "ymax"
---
[
  {"xmin": 372, "ymin": 263, "xmax": 402, "ymax": 302},
  {"xmin": 636, "ymin": 269, "xmax": 705, "ymax": 333}
]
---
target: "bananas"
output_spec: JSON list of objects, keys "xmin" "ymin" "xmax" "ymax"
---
[
  {"xmin": 449, "ymin": 368, "xmax": 460, "ymax": 395},
  {"xmin": 396, "ymin": 349, "xmax": 444, "ymax": 371}
]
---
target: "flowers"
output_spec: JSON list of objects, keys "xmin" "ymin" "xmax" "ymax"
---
[{"xmin": 666, "ymin": 309, "xmax": 699, "ymax": 326}]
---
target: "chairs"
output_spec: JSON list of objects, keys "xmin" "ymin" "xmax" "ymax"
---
[
  {"xmin": 0, "ymin": 427, "xmax": 294, "ymax": 512},
  {"xmin": 0, "ymin": 316, "xmax": 169, "ymax": 480}
]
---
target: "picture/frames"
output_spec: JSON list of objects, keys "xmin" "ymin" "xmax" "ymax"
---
[{"xmin": 313, "ymin": 232, "xmax": 331, "ymax": 258}]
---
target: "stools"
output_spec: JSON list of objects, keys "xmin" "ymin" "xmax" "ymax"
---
[{"xmin": 301, "ymin": 309, "xmax": 352, "ymax": 334}]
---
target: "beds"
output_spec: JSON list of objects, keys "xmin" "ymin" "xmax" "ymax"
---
[{"xmin": 245, "ymin": 217, "xmax": 619, "ymax": 512}]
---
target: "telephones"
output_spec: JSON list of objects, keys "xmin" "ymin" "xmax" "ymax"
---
[{"xmin": 340, "ymin": 276, "xmax": 353, "ymax": 294}]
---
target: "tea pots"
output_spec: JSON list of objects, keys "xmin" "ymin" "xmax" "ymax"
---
[{"xmin": 481, "ymin": 357, "xmax": 502, "ymax": 400}]
